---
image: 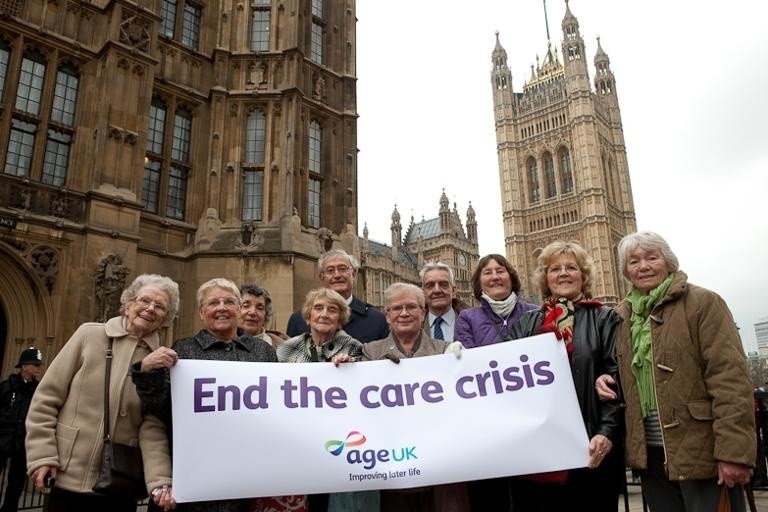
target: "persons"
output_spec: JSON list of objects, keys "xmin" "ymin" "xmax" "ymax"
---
[
  {"xmin": 361, "ymin": 283, "xmax": 451, "ymax": 512},
  {"xmin": 0, "ymin": 349, "xmax": 43, "ymax": 512},
  {"xmin": 236, "ymin": 284, "xmax": 290, "ymax": 350},
  {"xmin": 276, "ymin": 287, "xmax": 363, "ymax": 512},
  {"xmin": 502, "ymin": 241, "xmax": 623, "ymax": 511},
  {"xmin": 418, "ymin": 263, "xmax": 472, "ymax": 343},
  {"xmin": 25, "ymin": 274, "xmax": 176, "ymax": 512},
  {"xmin": 444, "ymin": 254, "xmax": 542, "ymax": 510},
  {"xmin": 286, "ymin": 249, "xmax": 390, "ymax": 344},
  {"xmin": 130, "ymin": 278, "xmax": 278, "ymax": 511},
  {"xmin": 595, "ymin": 231, "xmax": 757, "ymax": 512}
]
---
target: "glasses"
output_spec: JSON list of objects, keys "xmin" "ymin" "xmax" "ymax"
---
[
  {"xmin": 387, "ymin": 303, "xmax": 423, "ymax": 313},
  {"xmin": 128, "ymin": 295, "xmax": 165, "ymax": 313},
  {"xmin": 321, "ymin": 266, "xmax": 352, "ymax": 278}
]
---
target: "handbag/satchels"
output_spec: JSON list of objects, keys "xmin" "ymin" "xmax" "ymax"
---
[{"xmin": 92, "ymin": 439, "xmax": 149, "ymax": 501}]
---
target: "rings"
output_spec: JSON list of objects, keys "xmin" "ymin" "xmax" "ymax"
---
[{"xmin": 599, "ymin": 451, "xmax": 604, "ymax": 456}]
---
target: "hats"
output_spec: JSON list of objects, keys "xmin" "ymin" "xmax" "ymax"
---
[{"xmin": 14, "ymin": 346, "xmax": 42, "ymax": 368}]
